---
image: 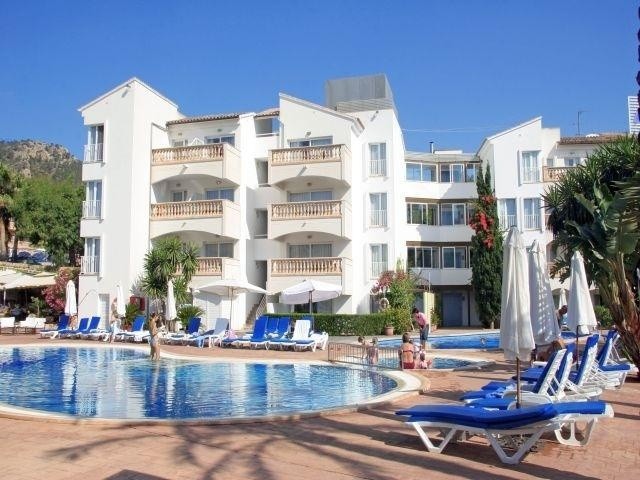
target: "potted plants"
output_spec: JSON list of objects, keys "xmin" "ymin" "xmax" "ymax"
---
[{"xmin": 383, "ymin": 306, "xmax": 394, "ymax": 337}]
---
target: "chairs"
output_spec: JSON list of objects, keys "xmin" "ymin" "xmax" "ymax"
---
[{"xmin": 395, "ymin": 328, "xmax": 640, "ymax": 465}]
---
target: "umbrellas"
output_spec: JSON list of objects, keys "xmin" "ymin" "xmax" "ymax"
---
[
  {"xmin": 64, "ymin": 280, "xmax": 77, "ymax": 327},
  {"xmin": 115, "ymin": 282, "xmax": 126, "ymax": 319},
  {"xmin": 278, "ymin": 278, "xmax": 343, "ymax": 314},
  {"xmin": 526, "ymin": 236, "xmax": 560, "ymax": 348},
  {"xmin": 564, "ymin": 248, "xmax": 597, "ymax": 372},
  {"xmin": 195, "ymin": 277, "xmax": 270, "ymax": 330},
  {"xmin": 165, "ymin": 280, "xmax": 177, "ymax": 321},
  {"xmin": 498, "ymin": 224, "xmax": 539, "ymax": 408}
]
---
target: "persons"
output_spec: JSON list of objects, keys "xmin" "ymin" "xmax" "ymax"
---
[
  {"xmin": 556, "ymin": 305, "xmax": 568, "ymax": 334},
  {"xmin": 396, "ymin": 332, "xmax": 430, "ymax": 369},
  {"xmin": 411, "ymin": 308, "xmax": 430, "ymax": 349},
  {"xmin": 367, "ymin": 337, "xmax": 381, "ymax": 364},
  {"xmin": 359, "ymin": 336, "xmax": 369, "ymax": 359},
  {"xmin": 148, "ymin": 312, "xmax": 166, "ymax": 361}
]
